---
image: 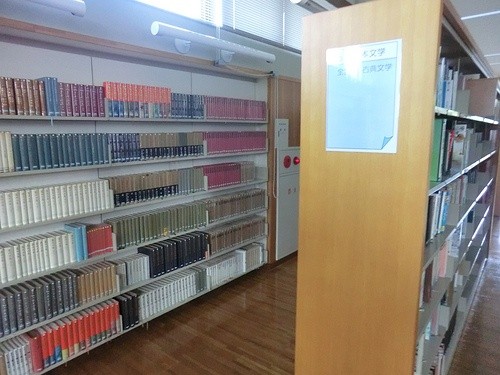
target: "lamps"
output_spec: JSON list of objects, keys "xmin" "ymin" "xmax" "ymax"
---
[
  {"xmin": 28, "ymin": 0, "xmax": 86, "ymax": 16},
  {"xmin": 151, "ymin": 21, "xmax": 275, "ymax": 65},
  {"xmin": 291, "ymin": 0, "xmax": 326, "ymax": 14}
]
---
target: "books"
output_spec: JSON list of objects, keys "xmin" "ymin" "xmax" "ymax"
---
[
  {"xmin": 413, "ymin": 63, "xmax": 500, "ymax": 373},
  {"xmin": 0, "ymin": 75, "xmax": 267, "ymax": 375}
]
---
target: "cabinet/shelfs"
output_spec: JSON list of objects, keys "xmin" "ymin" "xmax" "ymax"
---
[
  {"xmin": 0, "ymin": 17, "xmax": 278, "ymax": 374},
  {"xmin": 295, "ymin": 1, "xmax": 500, "ymax": 375}
]
